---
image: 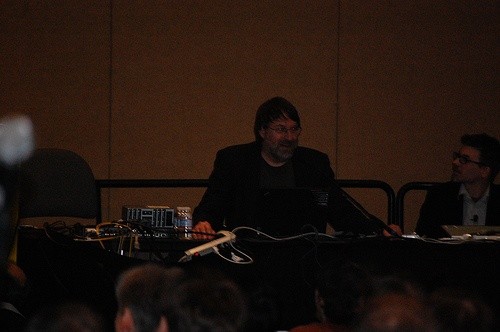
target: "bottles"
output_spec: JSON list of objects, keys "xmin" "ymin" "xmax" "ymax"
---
[{"xmin": 174, "ymin": 207, "xmax": 193, "ymax": 238}]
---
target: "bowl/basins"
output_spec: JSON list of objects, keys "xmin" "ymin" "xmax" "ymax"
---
[{"xmin": 443, "ymin": 225, "xmax": 500, "ymax": 238}]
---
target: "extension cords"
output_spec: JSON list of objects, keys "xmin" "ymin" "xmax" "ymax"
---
[{"xmin": 184, "ymin": 235, "xmax": 236, "ymax": 260}]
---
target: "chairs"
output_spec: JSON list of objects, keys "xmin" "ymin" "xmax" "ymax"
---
[{"xmin": 18, "ymin": 148, "xmax": 102, "ymax": 223}]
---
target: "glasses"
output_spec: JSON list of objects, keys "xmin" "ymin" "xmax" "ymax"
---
[
  {"xmin": 452, "ymin": 151, "xmax": 484, "ymax": 167},
  {"xmin": 267, "ymin": 125, "xmax": 303, "ymax": 136}
]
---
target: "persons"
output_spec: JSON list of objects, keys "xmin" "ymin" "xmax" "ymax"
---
[
  {"xmin": 31, "ymin": 264, "xmax": 245, "ymax": 332},
  {"xmin": 292, "ymin": 256, "xmax": 494, "ymax": 332},
  {"xmin": 193, "ymin": 97, "xmax": 403, "ymax": 332},
  {"xmin": 416, "ymin": 133, "xmax": 500, "ymax": 332}
]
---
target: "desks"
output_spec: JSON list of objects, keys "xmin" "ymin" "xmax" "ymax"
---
[{"xmin": 16, "ymin": 236, "xmax": 500, "ymax": 332}]
